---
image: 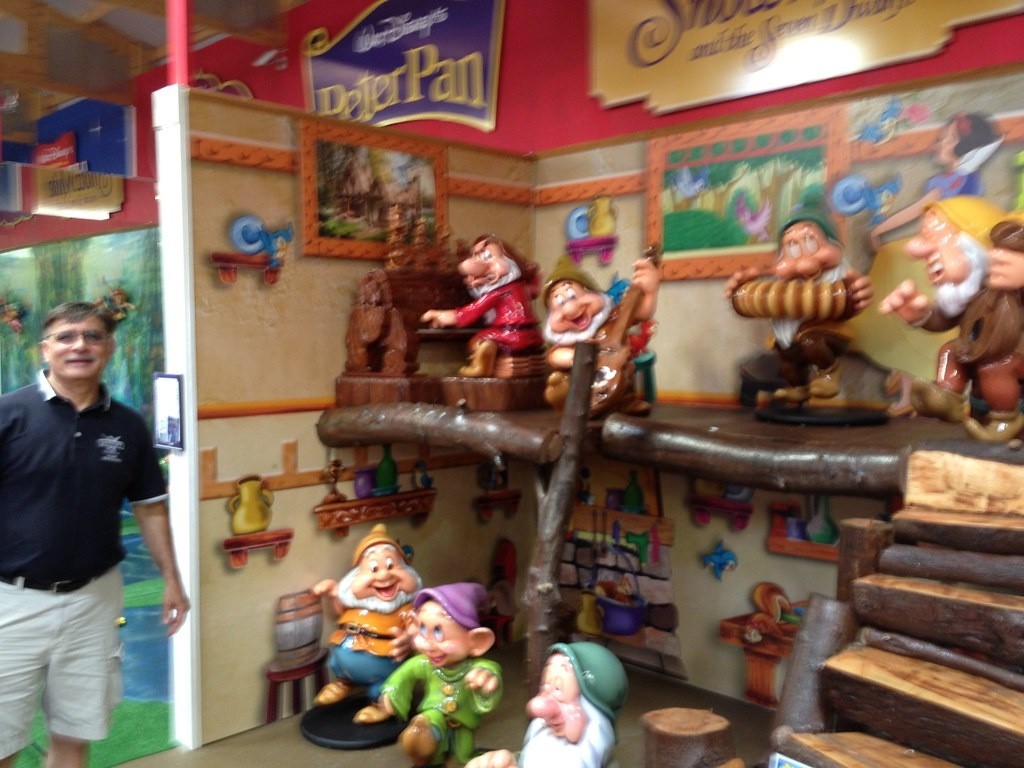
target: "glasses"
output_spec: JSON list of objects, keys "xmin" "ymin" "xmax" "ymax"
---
[{"xmin": 42, "ymin": 330, "xmax": 110, "ymax": 344}]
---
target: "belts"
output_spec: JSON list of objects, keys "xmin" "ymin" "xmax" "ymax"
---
[{"xmin": 0, "ymin": 577, "xmax": 92, "ymax": 593}]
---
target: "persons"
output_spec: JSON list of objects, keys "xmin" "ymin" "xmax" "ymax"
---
[
  {"xmin": 309, "ymin": 521, "xmax": 629, "ymax": 767},
  {"xmin": 1, "ymin": 300, "xmax": 191, "ymax": 767},
  {"xmin": 421, "ymin": 236, "xmax": 666, "ymax": 419},
  {"xmin": 719, "ymin": 184, "xmax": 1024, "ymax": 454}
]
